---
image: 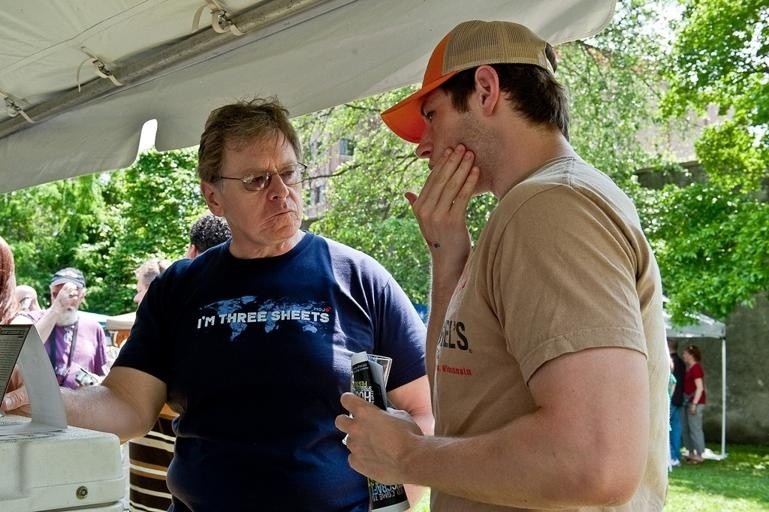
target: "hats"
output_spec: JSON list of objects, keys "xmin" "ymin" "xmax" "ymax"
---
[
  {"xmin": 379, "ymin": 20, "xmax": 558, "ymax": 145},
  {"xmin": 49, "ymin": 267, "xmax": 86, "ymax": 288}
]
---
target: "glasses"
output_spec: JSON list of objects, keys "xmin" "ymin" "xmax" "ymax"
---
[{"xmin": 220, "ymin": 161, "xmax": 308, "ymax": 192}]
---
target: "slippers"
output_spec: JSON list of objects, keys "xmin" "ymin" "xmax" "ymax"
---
[{"xmin": 670, "ymin": 457, "xmax": 706, "ymax": 467}]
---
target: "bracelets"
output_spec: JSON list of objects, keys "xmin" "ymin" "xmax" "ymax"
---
[{"xmin": 692, "ymin": 402, "xmax": 698, "ymax": 406}]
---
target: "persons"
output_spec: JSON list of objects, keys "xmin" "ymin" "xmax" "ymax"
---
[
  {"xmin": 134, "ymin": 258, "xmax": 172, "ymax": 306},
  {"xmin": 187, "ymin": 215, "xmax": 232, "ymax": 258},
  {"xmin": 666, "ymin": 337, "xmax": 686, "ymax": 468},
  {"xmin": 1, "ymin": 96, "xmax": 436, "ymax": 511},
  {"xmin": 681, "ymin": 344, "xmax": 706, "ymax": 465},
  {"xmin": 2, "ymin": 239, "xmax": 109, "ymax": 391},
  {"xmin": 334, "ymin": 19, "xmax": 673, "ymax": 512}
]
